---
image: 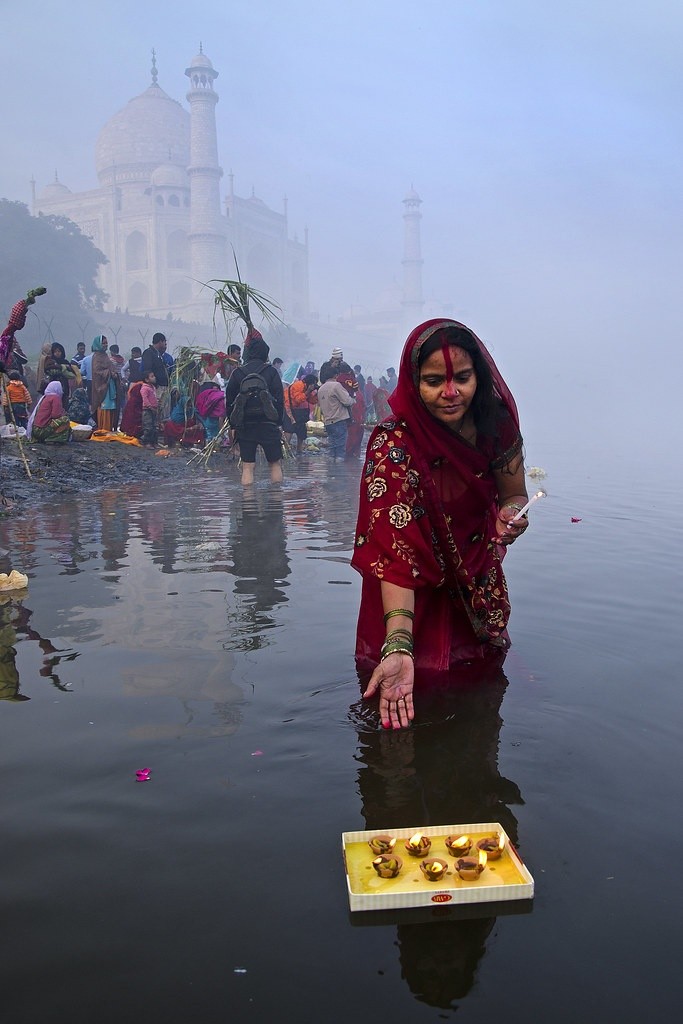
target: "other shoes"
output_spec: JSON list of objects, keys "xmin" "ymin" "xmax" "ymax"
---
[{"xmin": 144, "ymin": 442, "xmax": 162, "ymax": 450}]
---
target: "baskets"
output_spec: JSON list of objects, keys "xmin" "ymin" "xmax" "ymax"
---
[{"xmin": 69, "ymin": 429, "xmax": 93, "ymax": 442}]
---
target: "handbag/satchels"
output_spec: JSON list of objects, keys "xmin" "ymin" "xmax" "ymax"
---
[
  {"xmin": 291, "ymin": 408, "xmax": 310, "ymax": 423},
  {"xmin": 40, "ymin": 374, "xmax": 54, "ymax": 391},
  {"xmin": 0, "ymin": 423, "xmax": 27, "ymax": 440}
]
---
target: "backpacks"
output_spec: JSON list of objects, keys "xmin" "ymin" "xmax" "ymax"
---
[{"xmin": 228, "ymin": 363, "xmax": 283, "ymax": 430}]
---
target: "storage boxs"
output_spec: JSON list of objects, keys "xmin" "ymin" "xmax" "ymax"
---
[{"xmin": 341, "ymin": 822, "xmax": 535, "ymax": 912}]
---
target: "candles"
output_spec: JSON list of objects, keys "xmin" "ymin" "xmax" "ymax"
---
[
  {"xmin": 368, "ymin": 834, "xmax": 397, "ymax": 856},
  {"xmin": 475, "ymin": 832, "xmax": 506, "ymax": 862},
  {"xmin": 444, "ymin": 834, "xmax": 474, "ymax": 857},
  {"xmin": 454, "ymin": 849, "xmax": 487, "ymax": 882},
  {"xmin": 419, "ymin": 857, "xmax": 448, "ymax": 882},
  {"xmin": 372, "ymin": 854, "xmax": 403, "ymax": 879},
  {"xmin": 507, "ymin": 491, "xmax": 544, "ymax": 529},
  {"xmin": 404, "ymin": 831, "xmax": 432, "ymax": 858}
]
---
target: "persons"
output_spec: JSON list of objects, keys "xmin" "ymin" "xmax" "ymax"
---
[
  {"xmin": 0, "ymin": 332, "xmax": 398, "ymax": 464},
  {"xmin": 350, "ymin": 317, "xmax": 529, "ymax": 730},
  {"xmin": 225, "ymin": 337, "xmax": 285, "ymax": 485}
]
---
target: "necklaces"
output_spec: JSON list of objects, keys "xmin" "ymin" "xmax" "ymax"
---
[{"xmin": 457, "ymin": 414, "xmax": 465, "ymax": 433}]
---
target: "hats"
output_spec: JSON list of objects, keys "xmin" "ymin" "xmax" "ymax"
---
[
  {"xmin": 332, "ymin": 347, "xmax": 343, "ymax": 358},
  {"xmin": 7, "ymin": 368, "xmax": 22, "ymax": 380}
]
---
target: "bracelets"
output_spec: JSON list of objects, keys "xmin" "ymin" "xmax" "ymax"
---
[
  {"xmin": 380, "ymin": 608, "xmax": 414, "ymax": 661},
  {"xmin": 501, "ymin": 502, "xmax": 527, "ymax": 519}
]
